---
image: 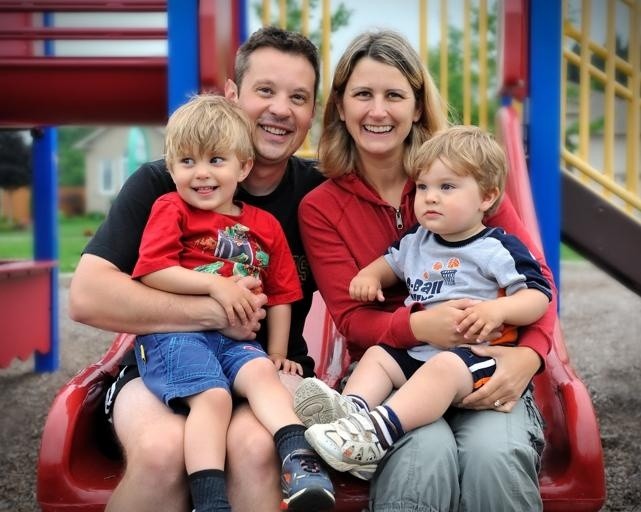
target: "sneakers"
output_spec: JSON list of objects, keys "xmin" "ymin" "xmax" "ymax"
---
[
  {"xmin": 302, "ymin": 407, "xmax": 388, "ymax": 482},
  {"xmin": 292, "ymin": 375, "xmax": 362, "ymax": 428},
  {"xmin": 278, "ymin": 448, "xmax": 337, "ymax": 512}
]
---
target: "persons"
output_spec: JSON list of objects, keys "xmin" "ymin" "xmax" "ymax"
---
[
  {"xmin": 69, "ymin": 27, "xmax": 332, "ymax": 512},
  {"xmin": 295, "ymin": 125, "xmax": 552, "ymax": 483},
  {"xmin": 132, "ymin": 94, "xmax": 334, "ymax": 510},
  {"xmin": 298, "ymin": 31, "xmax": 559, "ymax": 512}
]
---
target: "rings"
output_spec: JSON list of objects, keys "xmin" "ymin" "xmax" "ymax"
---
[{"xmin": 495, "ymin": 399, "xmax": 501, "ymax": 407}]
